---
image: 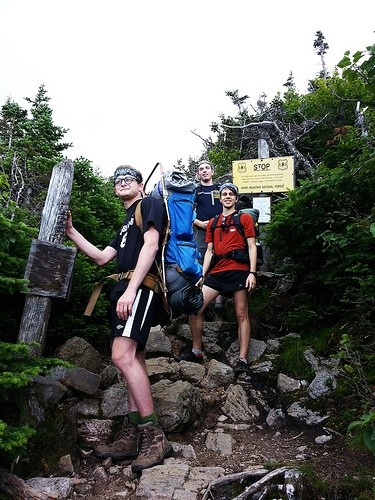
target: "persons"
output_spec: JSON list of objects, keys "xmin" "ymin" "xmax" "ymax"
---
[
  {"xmin": 174, "ymin": 183, "xmax": 258, "ymax": 372},
  {"xmin": 65, "ymin": 165, "xmax": 174, "ymax": 474},
  {"xmin": 192, "ymin": 160, "xmax": 224, "ymax": 309}
]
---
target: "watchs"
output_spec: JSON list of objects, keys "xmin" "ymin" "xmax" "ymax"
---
[{"xmin": 249, "ymin": 271, "xmax": 257, "ymax": 278}]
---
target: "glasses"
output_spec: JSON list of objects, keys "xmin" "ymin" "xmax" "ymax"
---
[{"xmin": 114, "ymin": 176, "xmax": 135, "ymax": 185}]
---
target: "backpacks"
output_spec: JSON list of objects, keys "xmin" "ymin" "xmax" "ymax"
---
[
  {"xmin": 135, "ymin": 170, "xmax": 203, "ymax": 279},
  {"xmin": 211, "ymin": 208, "xmax": 264, "ymax": 271}
]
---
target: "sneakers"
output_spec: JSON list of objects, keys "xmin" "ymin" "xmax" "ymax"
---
[
  {"xmin": 131, "ymin": 424, "xmax": 173, "ymax": 473},
  {"xmin": 95, "ymin": 426, "xmax": 139, "ymax": 459}
]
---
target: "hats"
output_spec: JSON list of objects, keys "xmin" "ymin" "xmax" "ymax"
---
[{"xmin": 114, "ymin": 165, "xmax": 143, "ymax": 183}]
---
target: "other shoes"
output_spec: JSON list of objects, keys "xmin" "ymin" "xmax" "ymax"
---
[
  {"xmin": 213, "ymin": 306, "xmax": 224, "ymax": 322},
  {"xmin": 174, "ymin": 350, "xmax": 204, "ymax": 364},
  {"xmin": 234, "ymin": 359, "xmax": 249, "ymax": 373}
]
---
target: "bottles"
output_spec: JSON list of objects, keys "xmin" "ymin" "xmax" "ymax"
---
[{"xmin": 255, "ymin": 222, "xmax": 258, "ymax": 232}]
